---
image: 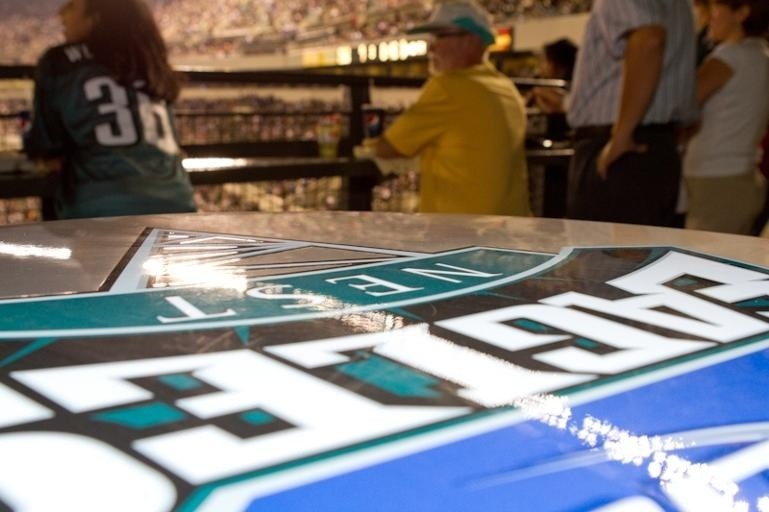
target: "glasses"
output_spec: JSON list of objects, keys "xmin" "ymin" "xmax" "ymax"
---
[{"xmin": 425, "ymin": 30, "xmax": 470, "ymax": 44}]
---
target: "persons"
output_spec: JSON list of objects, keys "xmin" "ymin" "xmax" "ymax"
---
[
  {"xmin": 21, "ymin": 1, "xmax": 196, "ymax": 220},
  {"xmin": 527, "ymin": 34, "xmax": 577, "ymax": 219},
  {"xmin": 561, "ymin": 0, "xmax": 699, "ymax": 229},
  {"xmin": 676, "ymin": 0, "xmax": 769, "ymax": 235},
  {"xmin": 353, "ymin": 1, "xmax": 536, "ymax": 218}
]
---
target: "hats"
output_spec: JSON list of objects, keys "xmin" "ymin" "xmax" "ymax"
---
[{"xmin": 404, "ymin": 0, "xmax": 497, "ymax": 46}]
